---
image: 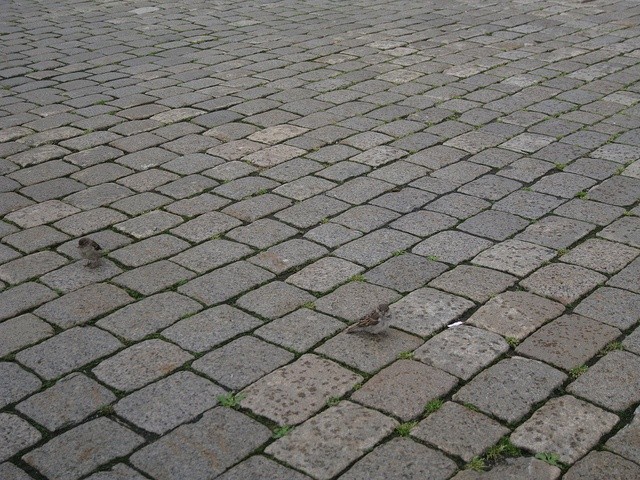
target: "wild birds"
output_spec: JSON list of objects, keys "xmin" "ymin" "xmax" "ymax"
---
[
  {"xmin": 345, "ymin": 303, "xmax": 393, "ymax": 340},
  {"xmin": 75, "ymin": 237, "xmax": 106, "ymax": 270}
]
